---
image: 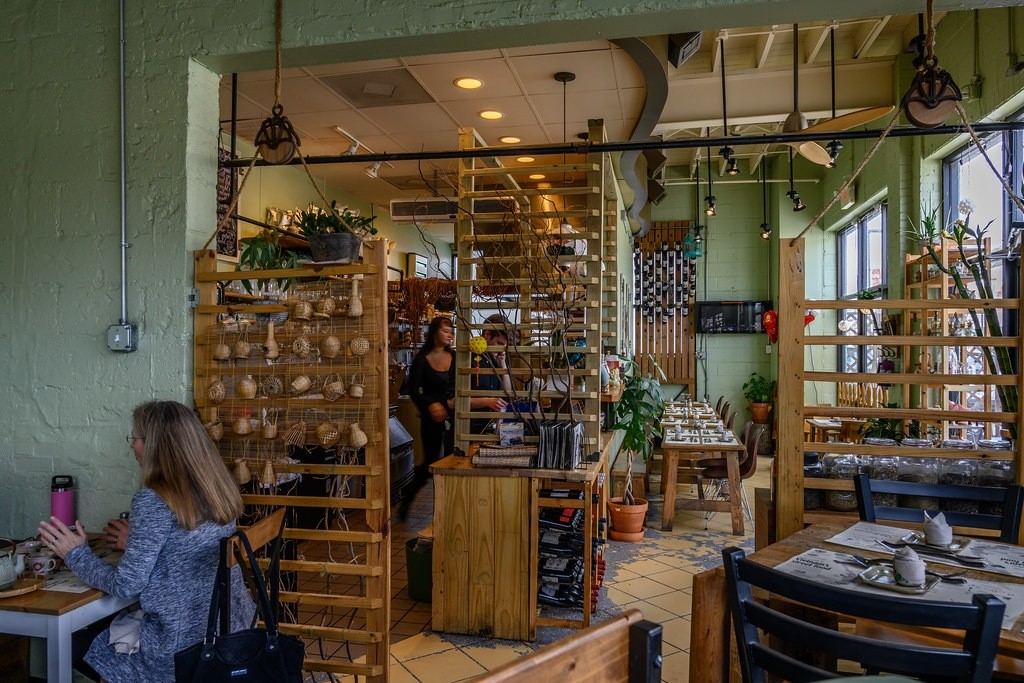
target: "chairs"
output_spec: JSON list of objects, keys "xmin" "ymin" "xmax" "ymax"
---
[
  {"xmin": 691, "ymin": 395, "xmax": 765, "ymax": 531},
  {"xmin": 853, "ymin": 474, "xmax": 1024, "ymax": 544},
  {"xmin": 721, "ymin": 546, "xmax": 1006, "ymax": 683},
  {"xmin": 174, "ymin": 505, "xmax": 290, "ymax": 683},
  {"xmin": 804, "ymin": 403, "xmax": 877, "ymax": 444}
]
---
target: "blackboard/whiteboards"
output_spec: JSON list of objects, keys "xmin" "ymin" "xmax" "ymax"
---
[{"xmin": 216, "ymin": 143, "xmax": 242, "ymax": 264}]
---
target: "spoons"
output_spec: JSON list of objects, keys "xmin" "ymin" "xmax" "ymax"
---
[
  {"xmin": 881, "ymin": 540, "xmax": 984, "ymax": 560},
  {"xmin": 852, "ymin": 555, "xmax": 968, "ymax": 584}
]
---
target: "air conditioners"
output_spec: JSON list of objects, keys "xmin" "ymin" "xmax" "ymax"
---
[{"xmin": 389, "ymin": 196, "xmax": 520, "ymax": 225}]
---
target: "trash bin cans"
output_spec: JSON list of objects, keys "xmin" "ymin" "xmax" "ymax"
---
[{"xmin": 404, "ymin": 538, "xmax": 432, "ymax": 603}]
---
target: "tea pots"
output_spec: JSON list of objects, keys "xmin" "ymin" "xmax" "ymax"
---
[{"xmin": 0, "ymin": 537, "xmax": 26, "ymax": 591}]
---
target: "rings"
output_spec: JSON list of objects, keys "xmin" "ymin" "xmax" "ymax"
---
[
  {"xmin": 115, "ymin": 537, "xmax": 117, "ymax": 542},
  {"xmin": 52, "ymin": 538, "xmax": 57, "ymax": 544}
]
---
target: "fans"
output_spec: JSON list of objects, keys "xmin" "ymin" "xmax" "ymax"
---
[{"xmin": 742, "ymin": 23, "xmax": 896, "ymax": 166}]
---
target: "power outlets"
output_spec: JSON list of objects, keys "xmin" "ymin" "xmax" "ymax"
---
[
  {"xmin": 106, "ymin": 325, "xmax": 137, "ymax": 352},
  {"xmin": 765, "ymin": 344, "xmax": 771, "ymax": 354}
]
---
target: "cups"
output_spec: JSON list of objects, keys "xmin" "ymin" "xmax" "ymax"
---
[
  {"xmin": 57, "ymin": 524, "xmax": 85, "ymax": 536},
  {"xmin": 16, "ymin": 539, "xmax": 56, "ymax": 579}
]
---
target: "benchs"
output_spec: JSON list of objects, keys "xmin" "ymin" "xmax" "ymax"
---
[{"xmin": 612, "ymin": 439, "xmax": 652, "ymax": 523}]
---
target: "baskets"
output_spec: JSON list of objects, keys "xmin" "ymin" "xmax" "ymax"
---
[
  {"xmin": 205, "ymin": 418, "xmax": 225, "ymax": 441},
  {"xmin": 292, "ymin": 334, "xmax": 312, "ymax": 360},
  {"xmin": 208, "ymin": 381, "xmax": 224, "ymax": 406},
  {"xmin": 314, "ymin": 411, "xmax": 339, "ymax": 449},
  {"xmin": 321, "ymin": 372, "xmax": 346, "ymax": 401},
  {"xmin": 350, "ymin": 335, "xmax": 370, "ymax": 357}
]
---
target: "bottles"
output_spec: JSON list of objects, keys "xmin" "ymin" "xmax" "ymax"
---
[
  {"xmin": 802, "ymin": 451, "xmax": 822, "ymax": 509},
  {"xmin": 119, "ymin": 511, "xmax": 129, "ymax": 521},
  {"xmin": 977, "ymin": 439, "xmax": 1014, "ymax": 515},
  {"xmin": 912, "ymin": 257, "xmax": 965, "ymax": 283},
  {"xmin": 860, "ymin": 437, "xmax": 899, "ymax": 507},
  {"xmin": 940, "ymin": 440, "xmax": 978, "ymax": 514},
  {"xmin": 823, "ymin": 441, "xmax": 860, "ymax": 511},
  {"xmin": 927, "ymin": 311, "xmax": 976, "ymax": 337},
  {"xmin": 634, "ymin": 241, "xmax": 696, "ymax": 324},
  {"xmin": 899, "ymin": 438, "xmax": 940, "ymax": 511}
]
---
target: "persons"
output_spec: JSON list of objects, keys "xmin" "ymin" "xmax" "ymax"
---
[
  {"xmin": 470, "ymin": 314, "xmax": 512, "ymax": 443},
  {"xmin": 397, "ymin": 317, "xmax": 456, "ymax": 523},
  {"xmin": 39, "ymin": 400, "xmax": 259, "ymax": 683}
]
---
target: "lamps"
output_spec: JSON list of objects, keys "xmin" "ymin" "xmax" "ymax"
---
[
  {"xmin": 718, "ymin": 40, "xmax": 742, "ymax": 176},
  {"xmin": 363, "ymin": 161, "xmax": 383, "ymax": 179},
  {"xmin": 785, "ymin": 145, "xmax": 807, "ymax": 212},
  {"xmin": 703, "ymin": 127, "xmax": 716, "ymax": 216},
  {"xmin": 370, "ymin": 202, "xmax": 378, "ymax": 236},
  {"xmin": 692, "ymin": 159, "xmax": 705, "ymax": 243},
  {"xmin": 759, "ymin": 156, "xmax": 772, "ymax": 240},
  {"xmin": 833, "ymin": 175, "xmax": 855, "ymax": 210},
  {"xmin": 546, "ymin": 71, "xmax": 580, "ymax": 241},
  {"xmin": 340, "ymin": 144, "xmax": 357, "ymax": 164},
  {"xmin": 825, "ymin": 27, "xmax": 844, "ymax": 168}
]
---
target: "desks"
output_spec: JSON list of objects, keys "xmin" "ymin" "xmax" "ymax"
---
[
  {"xmin": 715, "ymin": 523, "xmax": 1024, "ymax": 683},
  {"xmin": 806, "ymin": 419, "xmax": 858, "ymax": 442},
  {"xmin": 657, "ymin": 400, "xmax": 726, "ymax": 498},
  {"xmin": 660, "ymin": 426, "xmax": 745, "ymax": 537},
  {"xmin": 0, "ymin": 532, "xmax": 141, "ymax": 683}
]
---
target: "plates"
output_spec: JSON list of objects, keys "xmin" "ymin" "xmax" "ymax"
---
[
  {"xmin": 858, "ymin": 565, "xmax": 943, "ymax": 595},
  {"xmin": 665, "ymin": 402, "xmax": 733, "ymax": 442},
  {"xmin": 901, "ymin": 530, "xmax": 971, "ymax": 553}
]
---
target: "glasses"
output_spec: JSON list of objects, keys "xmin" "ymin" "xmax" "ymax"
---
[{"xmin": 126, "ymin": 435, "xmax": 146, "ymax": 443}]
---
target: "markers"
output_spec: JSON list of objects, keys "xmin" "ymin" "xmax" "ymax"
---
[{"xmin": 99, "ymin": 552, "xmax": 108, "ymax": 558}]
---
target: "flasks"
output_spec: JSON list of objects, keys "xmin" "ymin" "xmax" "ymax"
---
[{"xmin": 50, "ymin": 474, "xmax": 74, "ymax": 531}]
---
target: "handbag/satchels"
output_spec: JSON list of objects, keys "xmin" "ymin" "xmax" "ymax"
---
[{"xmin": 174, "ymin": 531, "xmax": 306, "ymax": 683}]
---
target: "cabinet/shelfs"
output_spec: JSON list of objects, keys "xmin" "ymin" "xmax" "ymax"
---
[
  {"xmin": 903, "ymin": 237, "xmax": 992, "ymax": 442},
  {"xmin": 428, "ymin": 426, "xmax": 625, "ymax": 642}
]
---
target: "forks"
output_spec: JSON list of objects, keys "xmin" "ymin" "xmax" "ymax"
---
[
  {"xmin": 874, "ymin": 539, "xmax": 986, "ymax": 568},
  {"xmin": 834, "ymin": 558, "xmax": 967, "ymax": 578}
]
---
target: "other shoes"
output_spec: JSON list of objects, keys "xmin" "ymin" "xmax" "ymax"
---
[{"xmin": 398, "ymin": 484, "xmax": 415, "ymax": 520}]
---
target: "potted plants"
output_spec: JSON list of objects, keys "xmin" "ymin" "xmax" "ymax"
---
[
  {"xmin": 293, "ymin": 200, "xmax": 376, "ymax": 263},
  {"xmin": 895, "ymin": 190, "xmax": 956, "ymax": 256},
  {"xmin": 742, "ymin": 372, "xmax": 777, "ymax": 424},
  {"xmin": 607, "ymin": 354, "xmax": 667, "ymax": 542},
  {"xmin": 236, "ymin": 227, "xmax": 312, "ymax": 325}
]
